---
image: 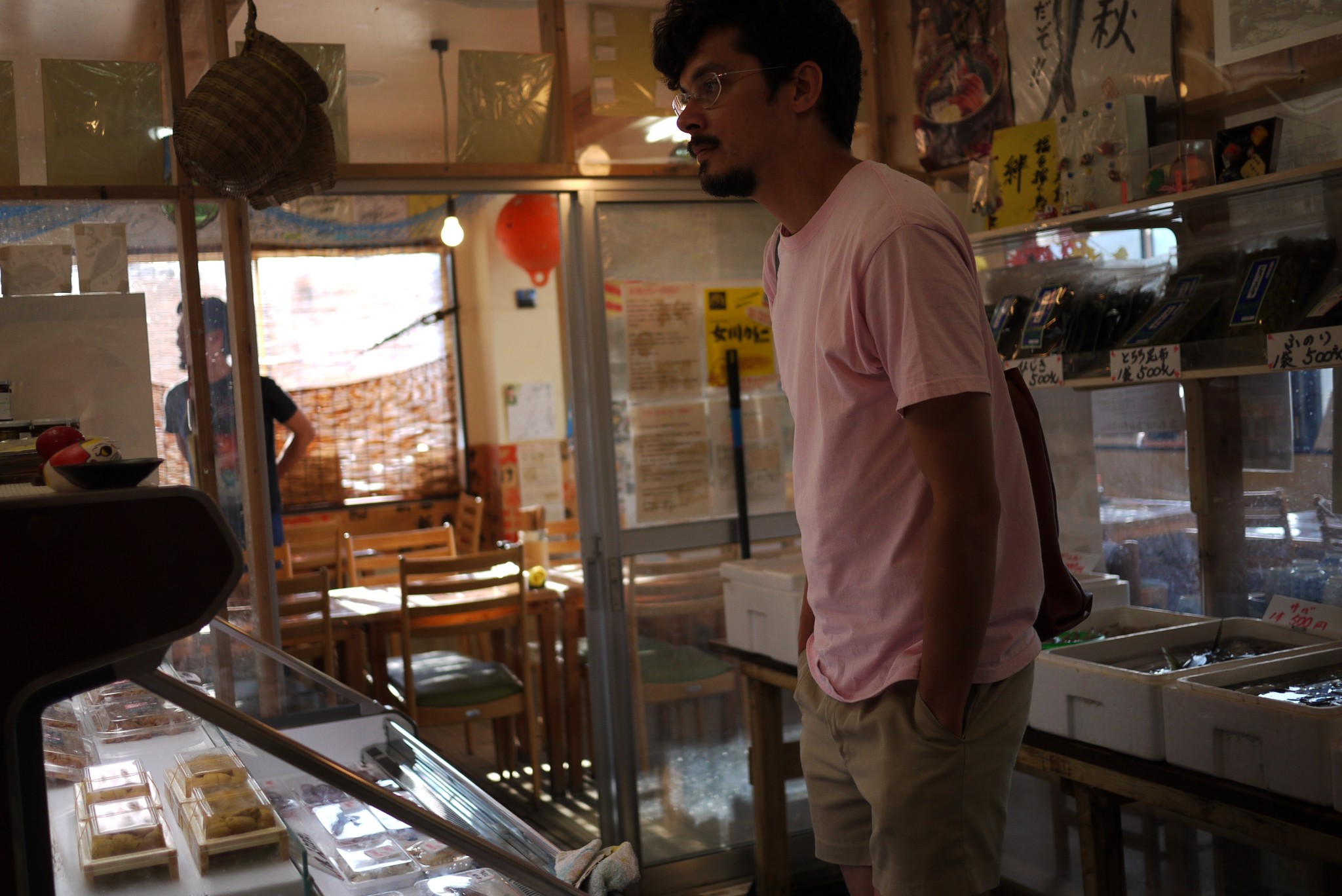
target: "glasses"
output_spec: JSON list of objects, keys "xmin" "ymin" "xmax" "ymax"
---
[{"xmin": 670, "ymin": 64, "xmax": 795, "ymax": 110}]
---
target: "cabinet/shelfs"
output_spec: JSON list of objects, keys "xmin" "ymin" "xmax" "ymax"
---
[
  {"xmin": 1, "ymin": 478, "xmax": 604, "ymax": 896},
  {"xmin": 969, "ymin": 157, "xmax": 1342, "ymax": 390}
]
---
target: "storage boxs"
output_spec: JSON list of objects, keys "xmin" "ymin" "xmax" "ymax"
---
[
  {"xmin": 1023, "ymin": 616, "xmax": 1327, "ymax": 780},
  {"xmin": 719, "ymin": 553, "xmax": 815, "ymax": 663},
  {"xmin": 1160, "ymin": 636, "xmax": 1342, "ymax": 829},
  {"xmin": 1040, "ymin": 603, "xmax": 1220, "ymax": 650}
]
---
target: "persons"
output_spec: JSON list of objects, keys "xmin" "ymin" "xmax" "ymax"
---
[
  {"xmin": 652, "ymin": 1, "xmax": 1052, "ymax": 896},
  {"xmin": 163, "ymin": 298, "xmax": 316, "ymax": 594}
]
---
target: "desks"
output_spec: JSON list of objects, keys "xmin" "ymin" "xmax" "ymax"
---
[
  {"xmin": 177, "ymin": 558, "xmax": 730, "ymax": 806},
  {"xmin": 704, "ymin": 637, "xmax": 1342, "ymax": 896},
  {"xmin": 1100, "ymin": 497, "xmax": 1195, "ymax": 540},
  {"xmin": 1186, "ymin": 510, "xmax": 1342, "ymax": 556}
]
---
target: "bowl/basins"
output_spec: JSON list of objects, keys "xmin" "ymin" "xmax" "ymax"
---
[{"xmin": 53, "ymin": 456, "xmax": 164, "ymax": 489}]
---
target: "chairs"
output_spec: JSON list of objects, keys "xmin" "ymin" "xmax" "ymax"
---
[
  {"xmin": 239, "ymin": 566, "xmax": 338, "ymax": 719},
  {"xmin": 518, "ymin": 500, "xmax": 680, "ymax": 800},
  {"xmin": 1102, "ymin": 541, "xmax": 1169, "ymax": 610},
  {"xmin": 377, "ymin": 539, "xmax": 545, "ymax": 814},
  {"xmin": 1313, "ymin": 494, "xmax": 1341, "ymax": 576},
  {"xmin": 340, "ymin": 522, "xmax": 513, "ymax": 786},
  {"xmin": 585, "ymin": 545, "xmax": 746, "ymax": 771},
  {"xmin": 1242, "ymin": 486, "xmax": 1296, "ymax": 566},
  {"xmin": 451, "ymin": 492, "xmax": 484, "ymax": 553}
]
